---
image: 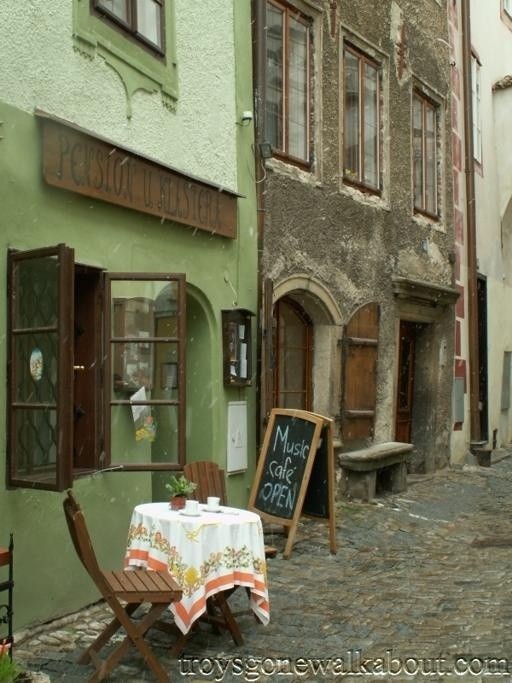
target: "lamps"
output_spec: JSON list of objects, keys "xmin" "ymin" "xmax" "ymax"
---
[{"xmin": 259, "ymin": 140, "xmax": 273, "ymax": 159}]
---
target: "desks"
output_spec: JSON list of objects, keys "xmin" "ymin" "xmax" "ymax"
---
[{"xmin": 130, "ymin": 501, "xmax": 264, "ymax": 648}]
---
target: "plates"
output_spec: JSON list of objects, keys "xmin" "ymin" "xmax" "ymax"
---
[
  {"xmin": 202, "ymin": 506, "xmax": 222, "ymax": 512},
  {"xmin": 180, "ymin": 509, "xmax": 202, "ymax": 517}
]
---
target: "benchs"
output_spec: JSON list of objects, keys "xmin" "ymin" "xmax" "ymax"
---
[{"xmin": 336, "ymin": 441, "xmax": 415, "ymax": 502}]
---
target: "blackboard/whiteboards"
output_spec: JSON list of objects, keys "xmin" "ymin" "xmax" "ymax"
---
[
  {"xmin": 249, "ymin": 408, "xmax": 324, "ymax": 527},
  {"xmin": 303, "ymin": 412, "xmax": 335, "ymax": 526}
]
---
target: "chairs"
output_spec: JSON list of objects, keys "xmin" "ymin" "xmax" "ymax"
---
[
  {"xmin": 181, "ymin": 461, "xmax": 279, "ymax": 634},
  {"xmin": 61, "ymin": 496, "xmax": 181, "ymax": 682}
]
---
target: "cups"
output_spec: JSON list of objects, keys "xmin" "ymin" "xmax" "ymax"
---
[
  {"xmin": 207, "ymin": 497, "xmax": 221, "ymax": 509},
  {"xmin": 186, "ymin": 500, "xmax": 200, "ymax": 513}
]
